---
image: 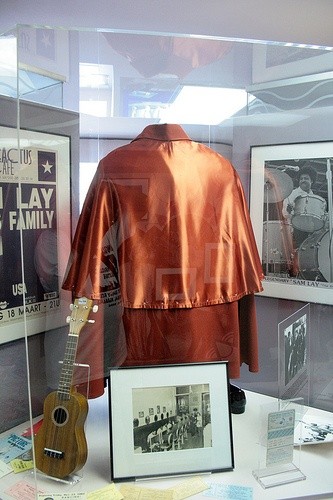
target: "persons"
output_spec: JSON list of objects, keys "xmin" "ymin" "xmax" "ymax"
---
[
  {"xmin": 103, "ymin": 190, "xmax": 179, "ymax": 367},
  {"xmin": 61, "ymin": 124, "xmax": 267, "ymax": 415},
  {"xmin": 280, "ymin": 168, "xmax": 315, "ymax": 278},
  {"xmin": 146, "ymin": 408, "xmax": 202, "ymax": 447},
  {"xmin": 33, "ymin": 173, "xmax": 130, "ymax": 399},
  {"xmin": 283, "ymin": 323, "xmax": 307, "ymax": 384}
]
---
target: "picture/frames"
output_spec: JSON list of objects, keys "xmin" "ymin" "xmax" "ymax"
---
[
  {"xmin": 244, "ymin": 139, "xmax": 333, "ymax": 306},
  {"xmin": 276, "ymin": 303, "xmax": 311, "ymax": 397},
  {"xmin": 0, "ymin": 124, "xmax": 75, "ymax": 347},
  {"xmin": 109, "ymin": 360, "xmax": 235, "ymax": 484}
]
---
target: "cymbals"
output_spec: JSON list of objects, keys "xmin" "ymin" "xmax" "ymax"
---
[{"xmin": 264, "ymin": 168, "xmax": 294, "ymax": 204}]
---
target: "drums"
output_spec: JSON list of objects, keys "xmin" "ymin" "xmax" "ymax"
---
[
  {"xmin": 291, "ymin": 194, "xmax": 327, "ymax": 232},
  {"xmin": 298, "ymin": 230, "xmax": 330, "ymax": 281},
  {"xmin": 262, "ymin": 220, "xmax": 294, "ymax": 265}
]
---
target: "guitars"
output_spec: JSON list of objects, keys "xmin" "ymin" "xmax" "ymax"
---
[{"xmin": 33, "ymin": 296, "xmax": 99, "ymax": 480}]
